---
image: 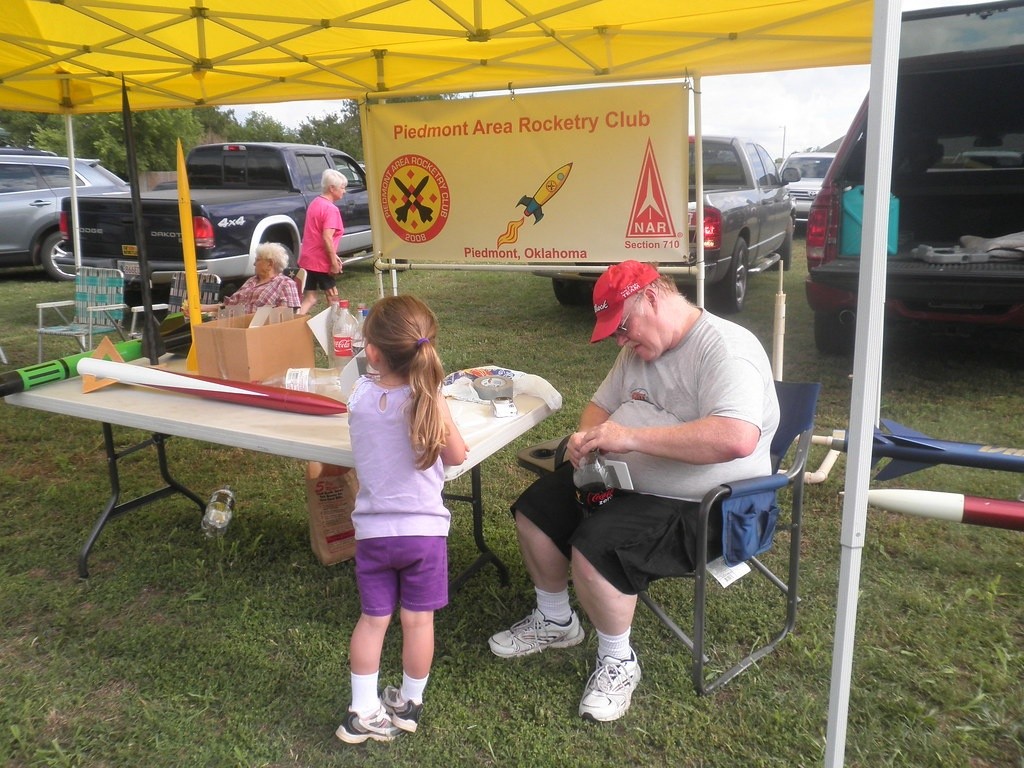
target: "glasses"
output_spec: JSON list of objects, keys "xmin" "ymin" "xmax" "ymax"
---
[{"xmin": 609, "ymin": 290, "xmax": 643, "ymax": 337}]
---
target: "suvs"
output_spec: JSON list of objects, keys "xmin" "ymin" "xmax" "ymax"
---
[
  {"xmin": 0, "ymin": 146, "xmax": 129, "ymax": 281},
  {"xmin": 779, "ymin": 150, "xmax": 837, "ymax": 228},
  {"xmin": 805, "ymin": 0, "xmax": 1024, "ymax": 354}
]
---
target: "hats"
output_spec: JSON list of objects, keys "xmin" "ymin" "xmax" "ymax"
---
[{"xmin": 590, "ymin": 259, "xmax": 660, "ymax": 344}]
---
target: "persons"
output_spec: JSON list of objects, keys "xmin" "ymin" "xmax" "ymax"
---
[
  {"xmin": 181, "ymin": 243, "xmax": 301, "ymax": 317},
  {"xmin": 488, "ymin": 260, "xmax": 781, "ymax": 722},
  {"xmin": 297, "ymin": 169, "xmax": 348, "ymax": 315},
  {"xmin": 335, "ymin": 294, "xmax": 469, "ymax": 744}
]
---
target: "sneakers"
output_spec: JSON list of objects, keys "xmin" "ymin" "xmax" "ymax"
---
[
  {"xmin": 579, "ymin": 646, "xmax": 640, "ymax": 720},
  {"xmin": 380, "ymin": 685, "xmax": 424, "ymax": 733},
  {"xmin": 488, "ymin": 606, "xmax": 585, "ymax": 659},
  {"xmin": 336, "ymin": 704, "xmax": 405, "ymax": 744}
]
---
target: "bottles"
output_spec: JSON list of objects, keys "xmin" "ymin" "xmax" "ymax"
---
[
  {"xmin": 182, "ymin": 299, "xmax": 190, "ymax": 323},
  {"xmin": 326, "ymin": 295, "xmax": 369, "ymax": 369},
  {"xmin": 202, "ymin": 486, "xmax": 236, "ymax": 536},
  {"xmin": 249, "ymin": 368, "xmax": 347, "ymax": 403},
  {"xmin": 573, "ymin": 447, "xmax": 612, "ymax": 521}
]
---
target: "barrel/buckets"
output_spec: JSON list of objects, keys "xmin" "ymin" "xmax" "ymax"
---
[{"xmin": 840, "ymin": 183, "xmax": 899, "ymax": 258}]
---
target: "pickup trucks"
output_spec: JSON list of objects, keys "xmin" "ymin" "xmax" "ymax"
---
[
  {"xmin": 54, "ymin": 141, "xmax": 408, "ymax": 311},
  {"xmin": 527, "ymin": 135, "xmax": 801, "ymax": 313}
]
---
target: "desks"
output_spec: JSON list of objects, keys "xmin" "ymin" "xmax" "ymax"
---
[{"xmin": 3, "ymin": 353, "xmax": 556, "ymax": 628}]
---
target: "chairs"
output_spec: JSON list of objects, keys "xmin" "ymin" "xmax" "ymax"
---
[
  {"xmin": 704, "ymin": 161, "xmax": 741, "ymax": 181},
  {"xmin": 35, "ymin": 265, "xmax": 129, "ymax": 364},
  {"xmin": 128, "ymin": 270, "xmax": 222, "ymax": 341},
  {"xmin": 552, "ymin": 379, "xmax": 820, "ymax": 697},
  {"xmin": 284, "ymin": 266, "xmax": 308, "ymax": 302}
]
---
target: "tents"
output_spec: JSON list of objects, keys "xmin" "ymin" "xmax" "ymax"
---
[{"xmin": 0, "ymin": 0, "xmax": 902, "ymax": 768}]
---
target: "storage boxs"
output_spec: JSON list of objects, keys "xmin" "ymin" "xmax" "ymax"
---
[{"xmin": 192, "ymin": 313, "xmax": 314, "ymax": 386}]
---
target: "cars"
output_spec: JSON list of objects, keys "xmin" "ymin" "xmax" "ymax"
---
[{"xmin": 338, "ymin": 162, "xmax": 367, "ymax": 182}]
